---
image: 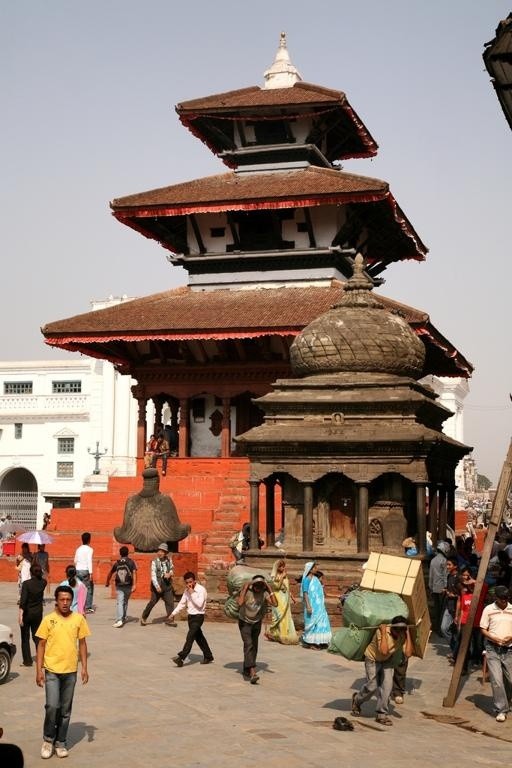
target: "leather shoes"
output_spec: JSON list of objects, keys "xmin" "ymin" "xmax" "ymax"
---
[
  {"xmin": 200, "ymin": 656, "xmax": 214, "ymax": 664},
  {"xmin": 171, "ymin": 656, "xmax": 183, "ymax": 667}
]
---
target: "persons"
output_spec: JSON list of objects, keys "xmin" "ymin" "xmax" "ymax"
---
[
  {"xmin": 167, "ymin": 572, "xmax": 215, "ymax": 667},
  {"xmin": 15, "ymin": 530, "xmax": 94, "ymax": 666},
  {"xmin": 1, "ymin": 511, "xmax": 52, "ymax": 556},
  {"xmin": 228, "ymin": 523, "xmax": 333, "ymax": 686},
  {"xmin": 144, "ymin": 423, "xmax": 181, "ymax": 476},
  {"xmin": 33, "ymin": 585, "xmax": 93, "ymax": 758},
  {"xmin": 141, "ymin": 543, "xmax": 178, "ymax": 627},
  {"xmin": 105, "ymin": 546, "xmax": 138, "ymax": 629},
  {"xmin": 342, "ymin": 510, "xmax": 512, "ymax": 725}
]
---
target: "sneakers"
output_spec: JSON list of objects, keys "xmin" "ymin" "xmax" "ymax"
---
[
  {"xmin": 113, "ymin": 619, "xmax": 126, "ymax": 628},
  {"xmin": 165, "ymin": 621, "xmax": 177, "ymax": 627},
  {"xmin": 395, "ymin": 695, "xmax": 403, "ymax": 704},
  {"xmin": 496, "ymin": 712, "xmax": 506, "ymax": 722},
  {"xmin": 40, "ymin": 739, "xmax": 53, "ymax": 759},
  {"xmin": 140, "ymin": 618, "xmax": 146, "ymax": 626},
  {"xmin": 250, "ymin": 674, "xmax": 259, "ymax": 684},
  {"xmin": 54, "ymin": 747, "xmax": 68, "ymax": 758}
]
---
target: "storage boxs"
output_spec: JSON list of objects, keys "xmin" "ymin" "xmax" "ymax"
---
[{"xmin": 359, "ymin": 551, "xmax": 432, "ymax": 660}]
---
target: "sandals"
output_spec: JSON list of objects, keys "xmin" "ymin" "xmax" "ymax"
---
[
  {"xmin": 376, "ymin": 714, "xmax": 393, "ymax": 726},
  {"xmin": 351, "ymin": 692, "xmax": 360, "ymax": 716}
]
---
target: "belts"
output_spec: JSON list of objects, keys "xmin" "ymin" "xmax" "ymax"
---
[{"xmin": 488, "ymin": 640, "xmax": 511, "ymax": 649}]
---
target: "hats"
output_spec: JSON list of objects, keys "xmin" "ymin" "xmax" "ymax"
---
[{"xmin": 156, "ymin": 543, "xmax": 169, "ymax": 552}]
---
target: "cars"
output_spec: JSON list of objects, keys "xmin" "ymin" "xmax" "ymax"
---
[{"xmin": 0, "ymin": 622, "xmax": 17, "ymax": 685}]
